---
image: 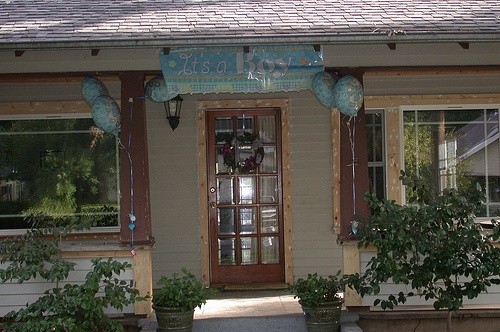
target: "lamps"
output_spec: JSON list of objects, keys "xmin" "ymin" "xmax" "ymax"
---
[{"xmin": 164, "ymin": 94, "xmax": 183, "ymax": 131}]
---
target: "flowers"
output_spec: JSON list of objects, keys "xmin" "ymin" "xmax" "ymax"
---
[{"xmin": 222, "ymin": 130, "xmax": 266, "ymax": 171}]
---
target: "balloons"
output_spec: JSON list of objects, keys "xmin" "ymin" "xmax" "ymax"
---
[
  {"xmin": 312, "ymin": 70, "xmax": 339, "ymax": 110},
  {"xmin": 81, "ymin": 76, "xmax": 109, "ymax": 108},
  {"xmin": 334, "ymin": 73, "xmax": 365, "ymax": 114},
  {"xmin": 92, "ymin": 95, "xmax": 121, "ymax": 137},
  {"xmin": 146, "ymin": 76, "xmax": 180, "ymax": 103}
]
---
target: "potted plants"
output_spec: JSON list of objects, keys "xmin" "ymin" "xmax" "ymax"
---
[
  {"xmin": 283, "ymin": 268, "xmax": 358, "ymax": 332},
  {"xmin": 152, "ymin": 268, "xmax": 208, "ymax": 332}
]
